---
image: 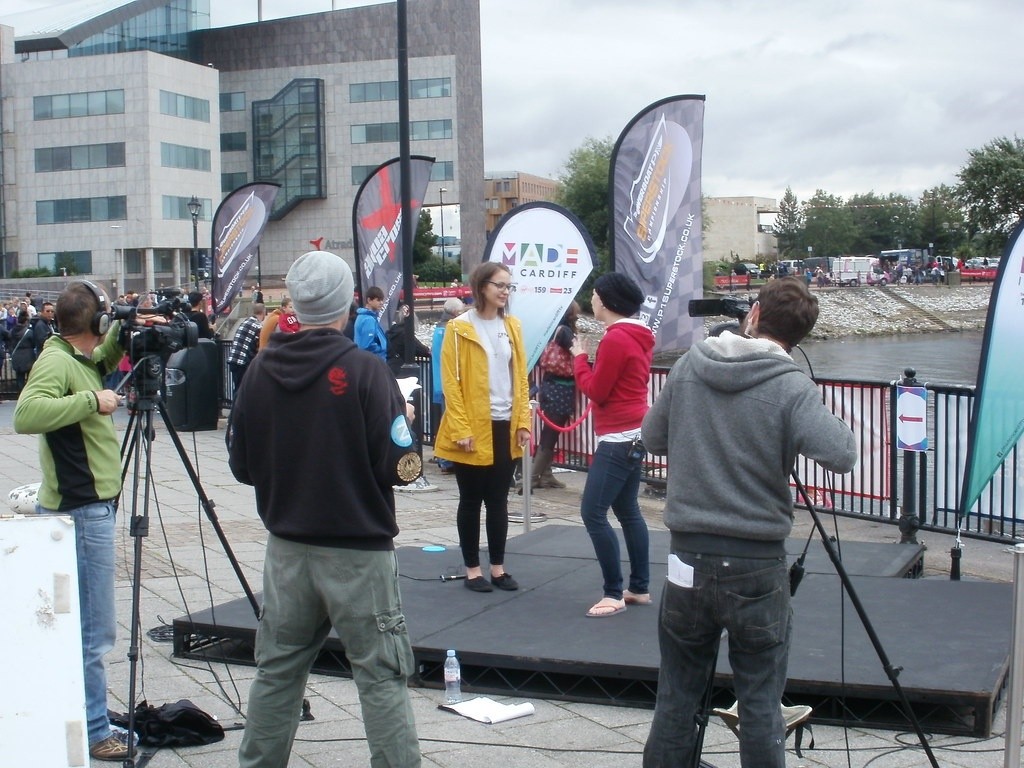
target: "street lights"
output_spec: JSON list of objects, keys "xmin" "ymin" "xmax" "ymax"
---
[
  {"xmin": 944, "ymin": 222, "xmax": 959, "ymax": 272},
  {"xmin": 111, "ymin": 225, "xmax": 126, "ymax": 294},
  {"xmin": 929, "ymin": 189, "xmax": 936, "ymax": 256},
  {"xmin": 790, "ymin": 221, "xmax": 806, "ymax": 274},
  {"xmin": 60, "ymin": 267, "xmax": 67, "ymax": 290},
  {"xmin": 187, "ymin": 195, "xmax": 201, "ymax": 291},
  {"xmin": 440, "ymin": 187, "xmax": 447, "ymax": 287}
]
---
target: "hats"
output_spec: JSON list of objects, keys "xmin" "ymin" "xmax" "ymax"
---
[
  {"xmin": 596, "ymin": 272, "xmax": 644, "ymax": 316},
  {"xmin": 441, "ymin": 298, "xmax": 464, "ymax": 319},
  {"xmin": 285, "ymin": 251, "xmax": 354, "ymax": 325}
]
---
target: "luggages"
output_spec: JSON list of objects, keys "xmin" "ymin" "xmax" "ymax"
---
[{"xmin": 900, "ymin": 276, "xmax": 907, "ymax": 284}]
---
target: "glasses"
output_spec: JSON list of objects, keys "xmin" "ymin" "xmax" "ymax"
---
[
  {"xmin": 46, "ymin": 310, "xmax": 54, "ymax": 313},
  {"xmin": 484, "ymin": 280, "xmax": 514, "ymax": 293},
  {"xmin": 111, "ymin": 306, "xmax": 116, "ymax": 320}
]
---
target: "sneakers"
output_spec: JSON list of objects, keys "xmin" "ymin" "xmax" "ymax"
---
[
  {"xmin": 89, "ymin": 735, "xmax": 138, "ymax": 760},
  {"xmin": 491, "ymin": 573, "xmax": 519, "ymax": 590},
  {"xmin": 464, "ymin": 576, "xmax": 494, "ymax": 592}
]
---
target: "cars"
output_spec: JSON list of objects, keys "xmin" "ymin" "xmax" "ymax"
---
[
  {"xmin": 936, "ymin": 256, "xmax": 1000, "ymax": 270},
  {"xmin": 735, "ymin": 263, "xmax": 760, "ymax": 277}
]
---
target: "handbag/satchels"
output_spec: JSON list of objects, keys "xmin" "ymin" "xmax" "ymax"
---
[
  {"xmin": 539, "ymin": 325, "xmax": 574, "ymax": 379},
  {"xmin": 931, "ymin": 271, "xmax": 936, "ymax": 275}
]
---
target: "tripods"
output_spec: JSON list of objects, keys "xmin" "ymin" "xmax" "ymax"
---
[{"xmin": 110, "ymin": 351, "xmax": 315, "ymax": 768}]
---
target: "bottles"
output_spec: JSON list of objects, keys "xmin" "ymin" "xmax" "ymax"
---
[{"xmin": 444, "ymin": 649, "xmax": 463, "ymax": 705}]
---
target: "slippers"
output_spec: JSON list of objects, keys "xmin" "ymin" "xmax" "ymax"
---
[
  {"xmin": 623, "ymin": 590, "xmax": 651, "ymax": 605},
  {"xmin": 585, "ymin": 606, "xmax": 627, "ymax": 618}
]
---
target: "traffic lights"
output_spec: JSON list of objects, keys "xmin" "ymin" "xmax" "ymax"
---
[{"xmin": 203, "ymin": 255, "xmax": 211, "ymax": 268}]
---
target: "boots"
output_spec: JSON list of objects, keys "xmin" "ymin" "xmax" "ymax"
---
[{"xmin": 530, "ymin": 445, "xmax": 566, "ymax": 489}]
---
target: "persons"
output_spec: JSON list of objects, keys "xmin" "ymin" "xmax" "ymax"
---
[
  {"xmin": 1, "ymin": 255, "xmax": 991, "ymax": 387},
  {"xmin": 226, "ymin": 251, "xmax": 423, "ymax": 768},
  {"xmin": 569, "ymin": 271, "xmax": 652, "ymax": 618},
  {"xmin": 429, "ymin": 297, "xmax": 465, "ymax": 471},
  {"xmin": 641, "ymin": 276, "xmax": 857, "ymax": 768},
  {"xmin": 432, "ymin": 263, "xmax": 530, "ymax": 593},
  {"xmin": 13, "ymin": 281, "xmax": 170, "ymax": 762},
  {"xmin": 529, "ymin": 298, "xmax": 576, "ymax": 491}
]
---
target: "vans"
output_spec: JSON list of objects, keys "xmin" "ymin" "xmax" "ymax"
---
[
  {"xmin": 780, "ymin": 260, "xmax": 804, "ymax": 274},
  {"xmin": 832, "ymin": 258, "xmax": 890, "ymax": 285}
]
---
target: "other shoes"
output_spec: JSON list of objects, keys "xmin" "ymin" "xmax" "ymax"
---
[{"xmin": 441, "ymin": 467, "xmax": 455, "ymax": 475}]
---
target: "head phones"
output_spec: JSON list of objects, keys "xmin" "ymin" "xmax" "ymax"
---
[{"xmin": 72, "ymin": 280, "xmax": 109, "ymax": 336}]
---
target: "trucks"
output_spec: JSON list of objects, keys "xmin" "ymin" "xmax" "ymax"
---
[
  {"xmin": 804, "ymin": 257, "xmax": 837, "ymax": 275},
  {"xmin": 882, "ymin": 250, "xmax": 937, "ymax": 278}
]
